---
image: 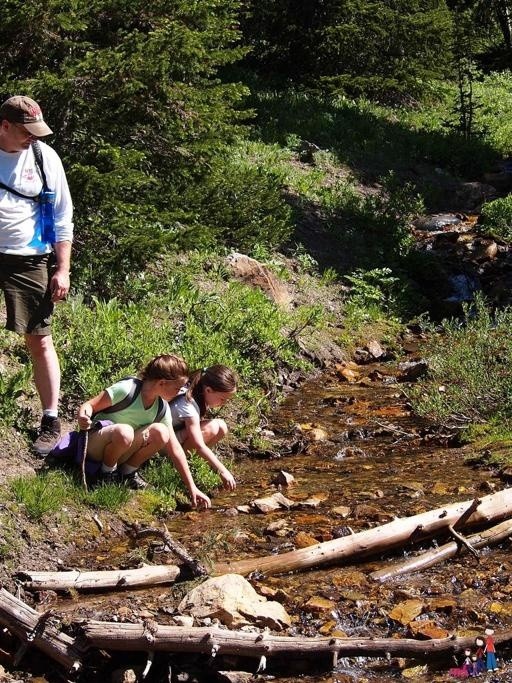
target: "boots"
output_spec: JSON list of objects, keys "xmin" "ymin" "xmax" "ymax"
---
[{"xmin": 31, "ymin": 416, "xmax": 61, "ymax": 456}]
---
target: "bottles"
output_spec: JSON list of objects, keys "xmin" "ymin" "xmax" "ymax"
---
[{"xmin": 40, "ymin": 190, "xmax": 57, "ymax": 242}]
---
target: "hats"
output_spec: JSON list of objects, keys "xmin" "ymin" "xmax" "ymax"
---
[{"xmin": 0, "ymin": 95, "xmax": 54, "ymax": 136}]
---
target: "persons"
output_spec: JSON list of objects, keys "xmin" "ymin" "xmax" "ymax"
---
[
  {"xmin": 77, "ymin": 355, "xmax": 212, "ymax": 510},
  {"xmin": 1, "ymin": 96, "xmax": 75, "ymax": 458},
  {"xmin": 158, "ymin": 364, "xmax": 236, "ymax": 492}
]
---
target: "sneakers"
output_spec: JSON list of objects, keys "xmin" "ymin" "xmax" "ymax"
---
[{"xmin": 93, "ymin": 466, "xmax": 155, "ymax": 490}]
